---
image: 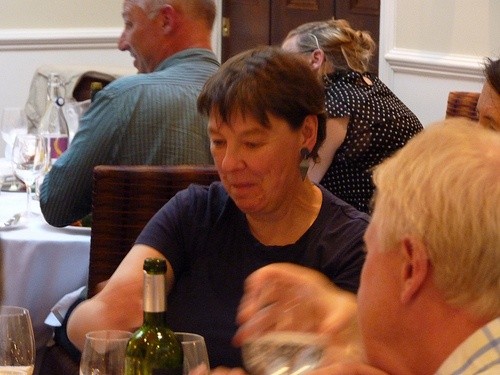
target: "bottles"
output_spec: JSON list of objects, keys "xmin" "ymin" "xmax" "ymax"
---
[
  {"xmin": 125, "ymin": 258, "xmax": 185, "ymax": 375},
  {"xmin": 37, "ymin": 73, "xmax": 69, "ymax": 175}
]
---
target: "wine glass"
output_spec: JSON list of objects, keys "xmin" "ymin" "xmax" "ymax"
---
[
  {"xmin": 0, "ymin": 107, "xmax": 29, "ymax": 192},
  {"xmin": 11, "ymin": 134, "xmax": 50, "ymax": 223}
]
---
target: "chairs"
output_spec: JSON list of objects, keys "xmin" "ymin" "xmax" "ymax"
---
[
  {"xmin": 88, "ymin": 164, "xmax": 222, "ymax": 304},
  {"xmin": 446, "ymin": 91, "xmax": 480, "ymax": 123}
]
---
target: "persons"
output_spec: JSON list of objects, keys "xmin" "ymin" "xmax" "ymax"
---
[
  {"xmin": 52, "ymin": 49, "xmax": 370, "ymax": 375},
  {"xmin": 283, "ymin": 19, "xmax": 424, "ymax": 213},
  {"xmin": 476, "ymin": 58, "xmax": 500, "ymax": 135},
  {"xmin": 231, "ymin": 117, "xmax": 500, "ymax": 375},
  {"xmin": 188, "ymin": 361, "xmax": 249, "ymax": 375},
  {"xmin": 40, "ymin": 0, "xmax": 222, "ymax": 229}
]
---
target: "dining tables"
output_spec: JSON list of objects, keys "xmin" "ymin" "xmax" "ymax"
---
[{"xmin": 0, "ymin": 192, "xmax": 91, "ymax": 350}]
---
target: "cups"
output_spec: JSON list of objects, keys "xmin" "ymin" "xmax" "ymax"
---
[
  {"xmin": 79, "ymin": 330, "xmax": 136, "ymax": 375},
  {"xmin": 0, "ymin": 306, "xmax": 35, "ymax": 375},
  {"xmin": 242, "ymin": 277, "xmax": 333, "ymax": 375},
  {"xmin": 173, "ymin": 333, "xmax": 210, "ymax": 375}
]
